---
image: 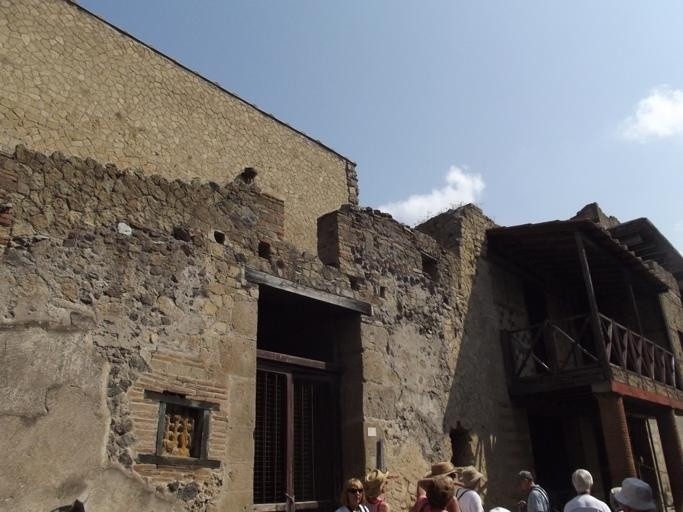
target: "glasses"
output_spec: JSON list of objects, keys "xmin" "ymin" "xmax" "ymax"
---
[
  {"xmin": 348, "ymin": 488, "xmax": 363, "ymax": 493},
  {"xmin": 448, "ymin": 474, "xmax": 455, "ymax": 478}
]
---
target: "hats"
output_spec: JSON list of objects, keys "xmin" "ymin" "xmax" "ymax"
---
[
  {"xmin": 610, "ymin": 477, "xmax": 656, "ymax": 510},
  {"xmin": 512, "ymin": 471, "xmax": 533, "ymax": 480},
  {"xmin": 462, "ymin": 466, "xmax": 484, "ymax": 488},
  {"xmin": 364, "ymin": 469, "xmax": 389, "ymax": 498},
  {"xmin": 423, "ymin": 462, "xmax": 464, "ymax": 480}
]
